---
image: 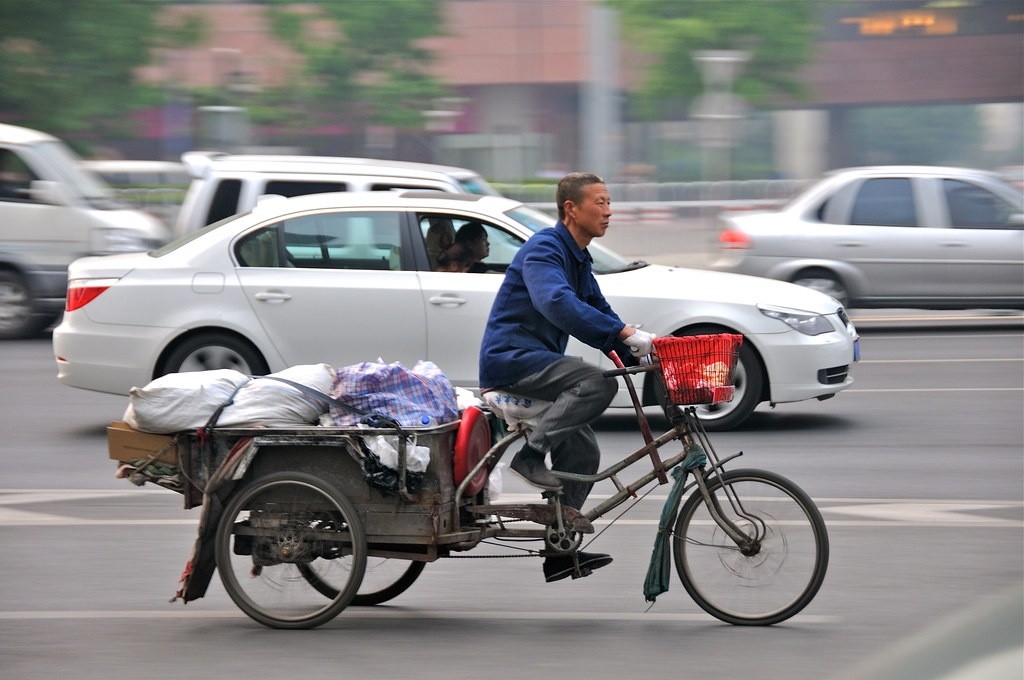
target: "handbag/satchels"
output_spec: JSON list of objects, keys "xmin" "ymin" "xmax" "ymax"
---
[{"xmin": 329, "ymin": 362, "xmax": 459, "ymax": 425}]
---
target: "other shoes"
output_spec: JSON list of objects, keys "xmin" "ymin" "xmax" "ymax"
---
[
  {"xmin": 543, "ymin": 552, "xmax": 613, "ymax": 582},
  {"xmin": 509, "ymin": 452, "xmax": 563, "ymax": 490}
]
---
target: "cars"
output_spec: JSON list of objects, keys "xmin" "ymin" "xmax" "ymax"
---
[
  {"xmin": 77, "ymin": 160, "xmax": 192, "ymax": 228},
  {"xmin": 51, "ymin": 184, "xmax": 863, "ymax": 432},
  {"xmin": 708, "ymin": 164, "xmax": 1024, "ymax": 313}
]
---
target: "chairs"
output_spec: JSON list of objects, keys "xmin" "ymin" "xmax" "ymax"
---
[{"xmin": 241, "ymin": 214, "xmax": 457, "ymax": 272}]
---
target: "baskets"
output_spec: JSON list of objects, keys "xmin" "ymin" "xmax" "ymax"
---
[{"xmin": 654, "ymin": 333, "xmax": 743, "ymax": 405}]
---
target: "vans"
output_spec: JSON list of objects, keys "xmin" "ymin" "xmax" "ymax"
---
[
  {"xmin": 0, "ymin": 118, "xmax": 172, "ymax": 342},
  {"xmin": 172, "ymin": 149, "xmax": 524, "ymax": 265}
]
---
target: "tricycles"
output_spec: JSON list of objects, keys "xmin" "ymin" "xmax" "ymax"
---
[{"xmin": 166, "ymin": 332, "xmax": 829, "ymax": 633}]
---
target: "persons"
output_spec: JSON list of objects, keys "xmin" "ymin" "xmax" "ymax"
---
[
  {"xmin": 478, "ymin": 172, "xmax": 657, "ymax": 585},
  {"xmin": 437, "ymin": 222, "xmax": 501, "ymax": 274}
]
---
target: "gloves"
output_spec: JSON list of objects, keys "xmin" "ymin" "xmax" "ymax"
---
[{"xmin": 622, "ymin": 329, "xmax": 653, "ymax": 357}]
